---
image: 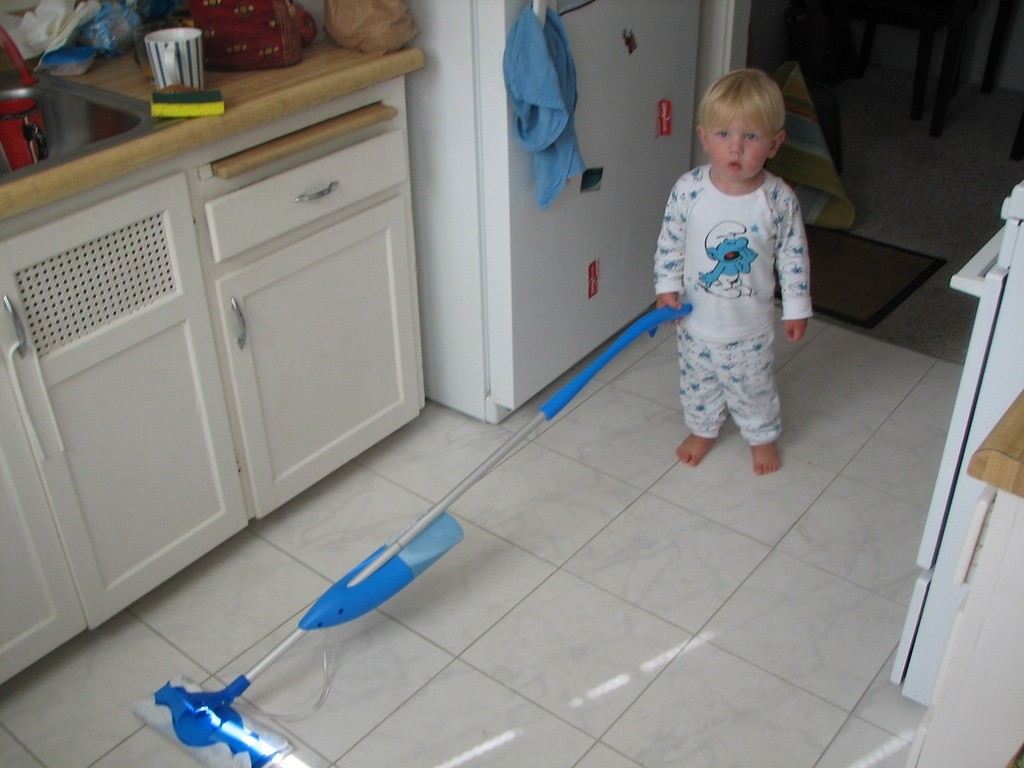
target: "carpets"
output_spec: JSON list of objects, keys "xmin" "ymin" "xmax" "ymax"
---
[{"xmin": 774, "ymin": 223, "xmax": 947, "ymax": 330}]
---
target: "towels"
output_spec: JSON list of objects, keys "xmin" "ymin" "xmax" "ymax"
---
[{"xmin": 503, "ymin": 2, "xmax": 587, "ymax": 208}]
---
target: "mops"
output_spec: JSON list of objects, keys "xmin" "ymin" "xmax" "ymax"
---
[{"xmin": 130, "ymin": 305, "xmax": 692, "ymax": 767}]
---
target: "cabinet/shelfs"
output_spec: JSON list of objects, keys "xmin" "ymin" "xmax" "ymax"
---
[
  {"xmin": 0, "ymin": 158, "xmax": 250, "ymax": 684},
  {"xmin": 180, "ymin": 76, "xmax": 426, "ymax": 522}
]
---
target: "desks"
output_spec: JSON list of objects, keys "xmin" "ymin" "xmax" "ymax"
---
[{"xmin": 787, "ymin": 0, "xmax": 1018, "ymax": 139}]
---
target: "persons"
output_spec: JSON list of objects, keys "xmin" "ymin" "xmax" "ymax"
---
[{"xmin": 653, "ymin": 68, "xmax": 815, "ymax": 476}]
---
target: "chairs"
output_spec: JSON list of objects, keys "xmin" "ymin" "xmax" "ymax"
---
[{"xmin": 843, "ymin": 0, "xmax": 979, "ymax": 121}]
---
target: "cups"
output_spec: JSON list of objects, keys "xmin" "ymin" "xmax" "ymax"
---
[
  {"xmin": 134, "ymin": 24, "xmax": 181, "ymax": 85},
  {"xmin": 144, "ymin": 27, "xmax": 204, "ymax": 94}
]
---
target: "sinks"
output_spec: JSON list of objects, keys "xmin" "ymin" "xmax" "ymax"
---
[{"xmin": 0, "ymin": 72, "xmax": 187, "ymax": 188}]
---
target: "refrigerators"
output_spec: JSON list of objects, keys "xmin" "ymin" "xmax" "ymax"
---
[{"xmin": 402, "ymin": 0, "xmax": 701, "ymax": 425}]
